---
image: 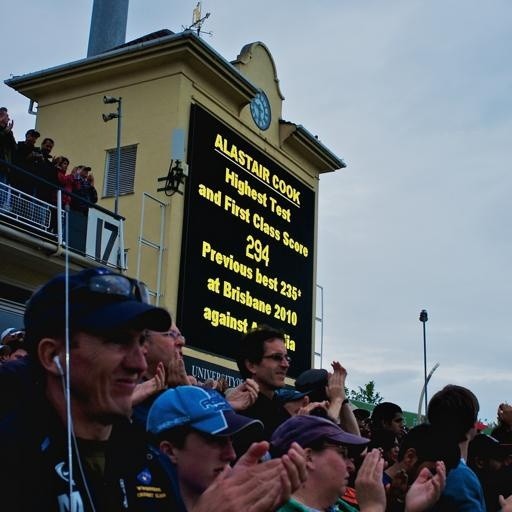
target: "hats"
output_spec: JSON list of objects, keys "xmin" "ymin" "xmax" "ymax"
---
[
  {"xmin": 23, "ymin": 269, "xmax": 172, "ymax": 334},
  {"xmin": 274, "ymin": 387, "xmax": 312, "ymax": 405},
  {"xmin": 295, "ymin": 370, "xmax": 329, "ymax": 399},
  {"xmin": 4, "ymin": 328, "xmax": 26, "ymax": 338},
  {"xmin": 143, "ymin": 385, "xmax": 266, "ymax": 438},
  {"xmin": 269, "ymin": 415, "xmax": 372, "ymax": 458}
]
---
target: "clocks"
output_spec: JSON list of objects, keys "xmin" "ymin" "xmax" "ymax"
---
[{"xmin": 250, "ymin": 89, "xmax": 272, "ymax": 130}]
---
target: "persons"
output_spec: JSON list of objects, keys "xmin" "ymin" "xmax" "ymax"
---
[
  {"xmin": 0, "ymin": 265, "xmax": 512, "ymax": 512},
  {"xmin": 0, "ymin": 107, "xmax": 98, "ymax": 233}
]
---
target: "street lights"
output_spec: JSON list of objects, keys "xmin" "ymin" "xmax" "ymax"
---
[
  {"xmin": 101, "ymin": 95, "xmax": 121, "ymax": 214},
  {"xmin": 420, "ymin": 310, "xmax": 429, "ymax": 416}
]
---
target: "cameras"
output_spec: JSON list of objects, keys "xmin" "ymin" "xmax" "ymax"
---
[{"xmin": 31, "ymin": 152, "xmax": 43, "ymax": 165}]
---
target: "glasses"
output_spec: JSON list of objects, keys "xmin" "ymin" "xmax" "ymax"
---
[
  {"xmin": 326, "ymin": 443, "xmax": 349, "ymax": 460},
  {"xmin": 69, "ymin": 272, "xmax": 150, "ymax": 307},
  {"xmin": 262, "ymin": 353, "xmax": 292, "ymax": 362}
]
